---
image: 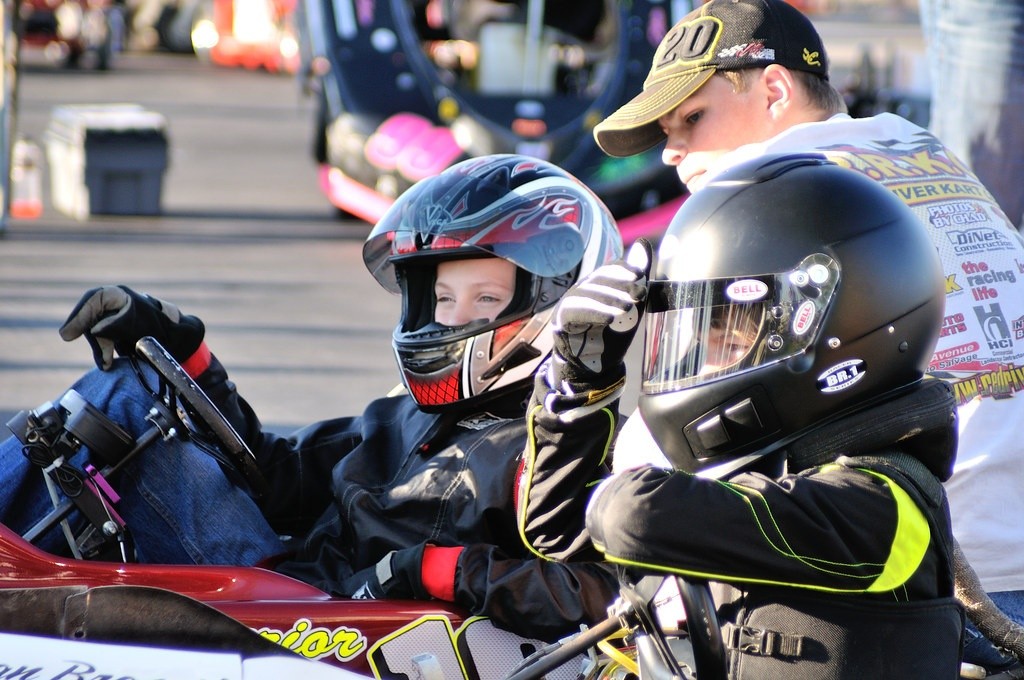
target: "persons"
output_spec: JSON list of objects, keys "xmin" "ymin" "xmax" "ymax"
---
[
  {"xmin": 56, "ymin": 0, "xmax": 110, "ymax": 70},
  {"xmin": 515, "ymin": 0, "xmax": 1024, "ymax": 668},
  {"xmin": 0, "ymin": 155, "xmax": 622, "ymax": 646}
]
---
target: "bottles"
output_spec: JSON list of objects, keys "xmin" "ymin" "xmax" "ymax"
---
[
  {"xmin": 11, "ymin": 135, "xmax": 43, "ymax": 172},
  {"xmin": 9, "ymin": 159, "xmax": 43, "ymax": 219}
]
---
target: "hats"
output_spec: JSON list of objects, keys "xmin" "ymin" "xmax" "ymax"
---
[{"xmin": 594, "ymin": 1, "xmax": 826, "ymax": 157}]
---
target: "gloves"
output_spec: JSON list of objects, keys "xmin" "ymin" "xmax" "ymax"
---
[
  {"xmin": 314, "ymin": 540, "xmax": 444, "ymax": 603},
  {"xmin": 58, "ymin": 285, "xmax": 205, "ymax": 371},
  {"xmin": 543, "ymin": 237, "xmax": 652, "ymax": 395}
]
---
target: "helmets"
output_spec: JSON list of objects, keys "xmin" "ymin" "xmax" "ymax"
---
[
  {"xmin": 638, "ymin": 152, "xmax": 944, "ymax": 475},
  {"xmin": 389, "ymin": 152, "xmax": 623, "ymax": 412}
]
